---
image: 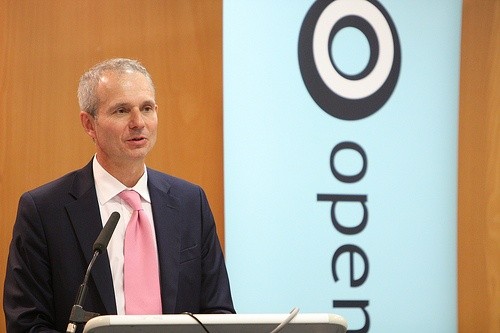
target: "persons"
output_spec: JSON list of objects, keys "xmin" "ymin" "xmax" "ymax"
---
[{"xmin": 3, "ymin": 58, "xmax": 236, "ymax": 333}]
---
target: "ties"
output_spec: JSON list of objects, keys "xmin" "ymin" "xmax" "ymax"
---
[{"xmin": 118, "ymin": 190, "xmax": 162, "ymax": 315}]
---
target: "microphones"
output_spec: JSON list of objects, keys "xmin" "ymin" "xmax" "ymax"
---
[{"xmin": 66, "ymin": 211, "xmax": 120, "ymax": 332}]
